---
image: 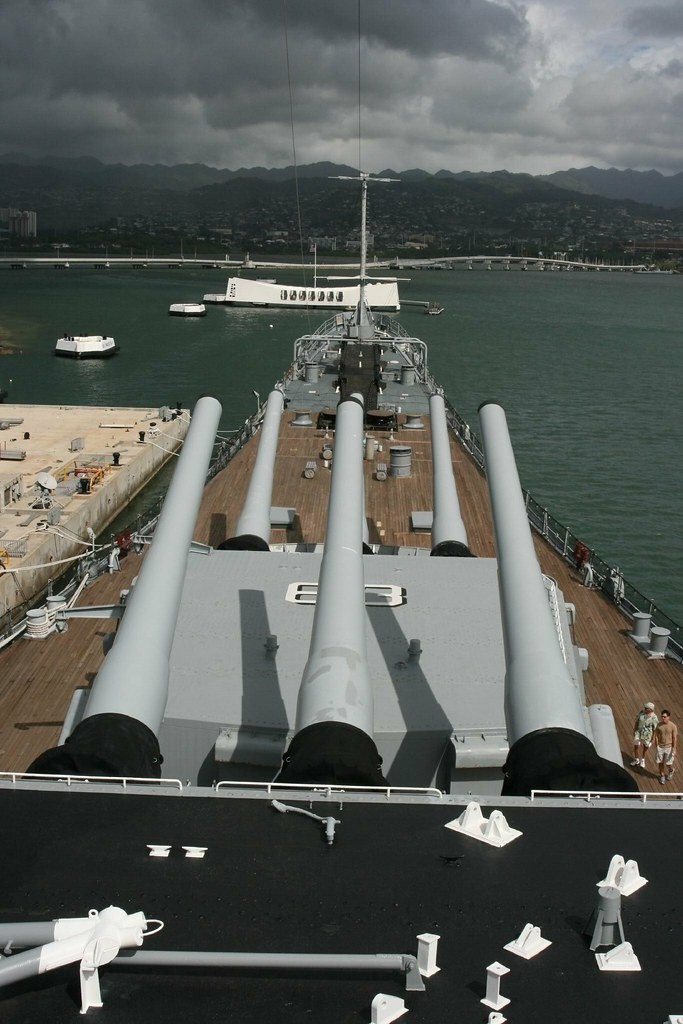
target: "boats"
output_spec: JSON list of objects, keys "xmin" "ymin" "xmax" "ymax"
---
[
  {"xmin": 203, "ymin": 274, "xmax": 400, "ymax": 313},
  {"xmin": 634, "ymin": 266, "xmax": 674, "ymax": 275},
  {"xmin": 54, "ymin": 335, "xmax": 120, "ymax": 360},
  {"xmin": 169, "ymin": 302, "xmax": 207, "ymax": 317}
]
